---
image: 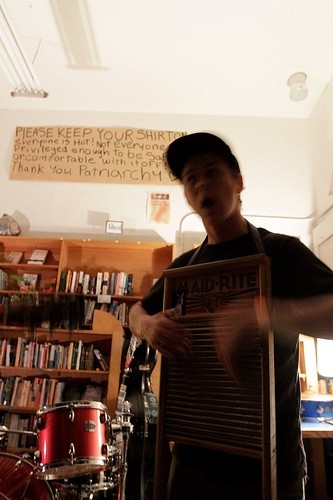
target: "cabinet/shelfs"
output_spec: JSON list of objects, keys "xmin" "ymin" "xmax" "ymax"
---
[{"xmin": 0, "ymin": 235, "xmax": 176, "ymax": 463}]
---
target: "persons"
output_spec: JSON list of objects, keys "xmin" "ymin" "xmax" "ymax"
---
[{"xmin": 126, "ymin": 132, "xmax": 333, "ymax": 500}]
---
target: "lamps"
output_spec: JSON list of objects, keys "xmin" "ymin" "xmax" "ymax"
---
[
  {"xmin": 0, "ymin": 2, "xmax": 49, "ymax": 98},
  {"xmin": 288, "ymin": 73, "xmax": 308, "ymax": 101}
]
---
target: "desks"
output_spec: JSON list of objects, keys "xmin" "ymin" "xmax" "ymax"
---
[{"xmin": 301, "ymin": 420, "xmax": 333, "ymax": 500}]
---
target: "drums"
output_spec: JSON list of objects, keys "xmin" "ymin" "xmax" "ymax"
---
[
  {"xmin": 0, "ymin": 445, "xmax": 79, "ymax": 500},
  {"xmin": 52, "ymin": 443, "xmax": 121, "ymax": 491},
  {"xmin": 32, "ymin": 400, "xmax": 112, "ymax": 480}
]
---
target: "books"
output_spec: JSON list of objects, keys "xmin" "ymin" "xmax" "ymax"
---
[
  {"xmin": 0, "ymin": 339, "xmax": 109, "ymax": 372},
  {"xmin": 0, "ymin": 298, "xmax": 129, "ymax": 326},
  {"xmin": 0, "ymin": 377, "xmax": 103, "ymax": 409},
  {"xmin": 59, "ymin": 271, "xmax": 134, "ymax": 296},
  {"xmin": 0, "ymin": 413, "xmax": 35, "ymax": 449},
  {"xmin": 0, "ymin": 270, "xmax": 9, "ymax": 290},
  {"xmin": 5, "ymin": 250, "xmax": 23, "ymax": 264},
  {"xmin": 27, "ymin": 249, "xmax": 48, "ymax": 265},
  {"xmin": 21, "ymin": 274, "xmax": 38, "ymax": 292}
]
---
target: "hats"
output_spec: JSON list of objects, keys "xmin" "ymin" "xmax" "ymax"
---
[{"xmin": 166, "ymin": 132, "xmax": 241, "ymax": 182}]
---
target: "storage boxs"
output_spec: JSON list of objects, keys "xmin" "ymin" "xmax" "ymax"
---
[{"xmin": 301, "ymin": 399, "xmax": 333, "ymax": 421}]
